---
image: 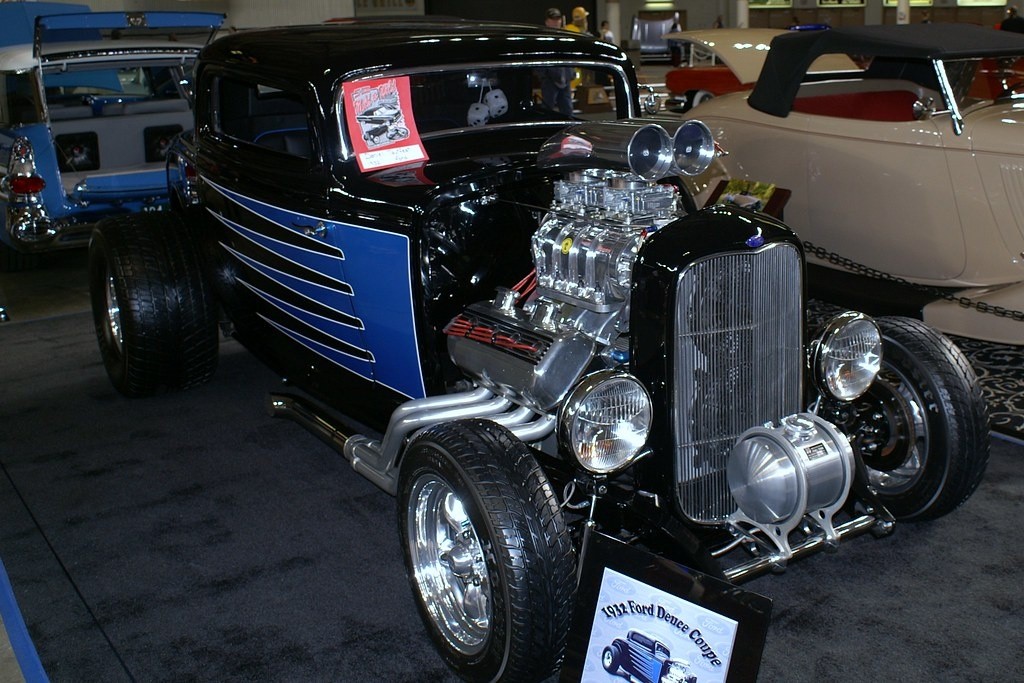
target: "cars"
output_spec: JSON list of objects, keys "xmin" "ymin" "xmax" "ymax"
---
[
  {"xmin": 356, "ymin": 107, "xmax": 407, "ymax": 145},
  {"xmin": 601, "ymin": 627, "xmax": 700, "ymax": 683},
  {"xmin": 0, "ymin": 11, "xmax": 311, "ymax": 275},
  {"xmin": 631, "ymin": 6, "xmax": 1023, "ymax": 347},
  {"xmin": 88, "ymin": 14, "xmax": 994, "ymax": 683}
]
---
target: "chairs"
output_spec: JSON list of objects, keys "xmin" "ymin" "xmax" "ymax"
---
[
  {"xmin": 124, "ymin": 99, "xmax": 190, "ymax": 115},
  {"xmin": 25, "ymin": 104, "xmax": 94, "ymax": 121}
]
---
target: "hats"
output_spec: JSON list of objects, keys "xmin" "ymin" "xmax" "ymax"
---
[
  {"xmin": 571, "ymin": 6, "xmax": 590, "ymax": 20},
  {"xmin": 545, "ymin": 7, "xmax": 562, "ymax": 18}
]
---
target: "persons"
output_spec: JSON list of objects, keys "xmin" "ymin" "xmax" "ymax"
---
[
  {"xmin": 999, "ymin": 5, "xmax": 1024, "ymax": 35},
  {"xmin": 920, "ymin": 9, "xmax": 932, "ymax": 22},
  {"xmin": 533, "ymin": 9, "xmax": 577, "ymax": 117},
  {"xmin": 562, "ymin": 8, "xmax": 724, "ymax": 100},
  {"xmin": 784, "ymin": 17, "xmax": 800, "ymax": 29}
]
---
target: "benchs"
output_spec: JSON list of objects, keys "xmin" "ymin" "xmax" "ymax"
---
[
  {"xmin": 254, "ymin": 119, "xmax": 461, "ymax": 159},
  {"xmin": 793, "ymin": 91, "xmax": 919, "ymax": 121}
]
